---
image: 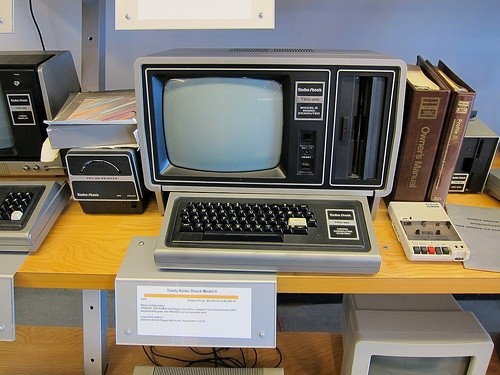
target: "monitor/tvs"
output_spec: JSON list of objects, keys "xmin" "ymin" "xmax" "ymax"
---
[
  {"xmin": 0, "ymin": 50, "xmax": 82, "ymax": 176},
  {"xmin": 134, "ymin": 47, "xmax": 407, "ymax": 218}
]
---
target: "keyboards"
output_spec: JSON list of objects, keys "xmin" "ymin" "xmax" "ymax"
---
[
  {"xmin": 153, "ymin": 193, "xmax": 381, "ymax": 275},
  {"xmin": 0, "ymin": 181, "xmax": 73, "ymax": 252}
]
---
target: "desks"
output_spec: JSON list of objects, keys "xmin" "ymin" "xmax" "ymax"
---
[{"xmin": 0, "ymin": 146, "xmax": 500, "ymax": 375}]
